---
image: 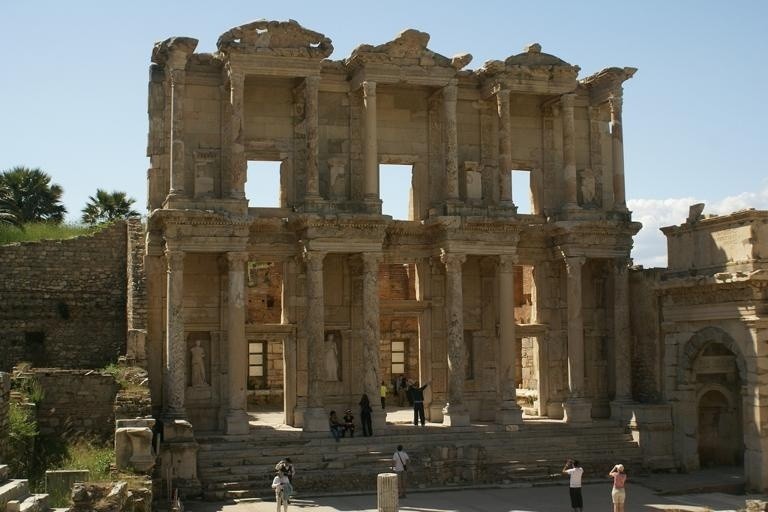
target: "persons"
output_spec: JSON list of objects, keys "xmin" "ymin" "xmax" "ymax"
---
[
  {"xmin": 392, "ymin": 445, "xmax": 409, "ymax": 498},
  {"xmin": 562, "ymin": 459, "xmax": 584, "ymax": 512},
  {"xmin": 609, "ymin": 464, "xmax": 627, "ymax": 512},
  {"xmin": 325, "ymin": 334, "xmax": 338, "ymax": 381},
  {"xmin": 272, "ymin": 469, "xmax": 289, "ymax": 512},
  {"xmin": 275, "ymin": 457, "xmax": 295, "ymax": 505},
  {"xmin": 190, "ymin": 340, "xmax": 209, "ymax": 388},
  {"xmin": 329, "ymin": 374, "xmax": 427, "ymax": 442}
]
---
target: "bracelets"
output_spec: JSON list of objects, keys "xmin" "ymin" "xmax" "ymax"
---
[{"xmin": 566, "ymin": 464, "xmax": 568, "ymax": 467}]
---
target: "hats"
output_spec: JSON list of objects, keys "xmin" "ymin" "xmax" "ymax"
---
[{"xmin": 615, "ymin": 464, "xmax": 624, "ymax": 471}]
